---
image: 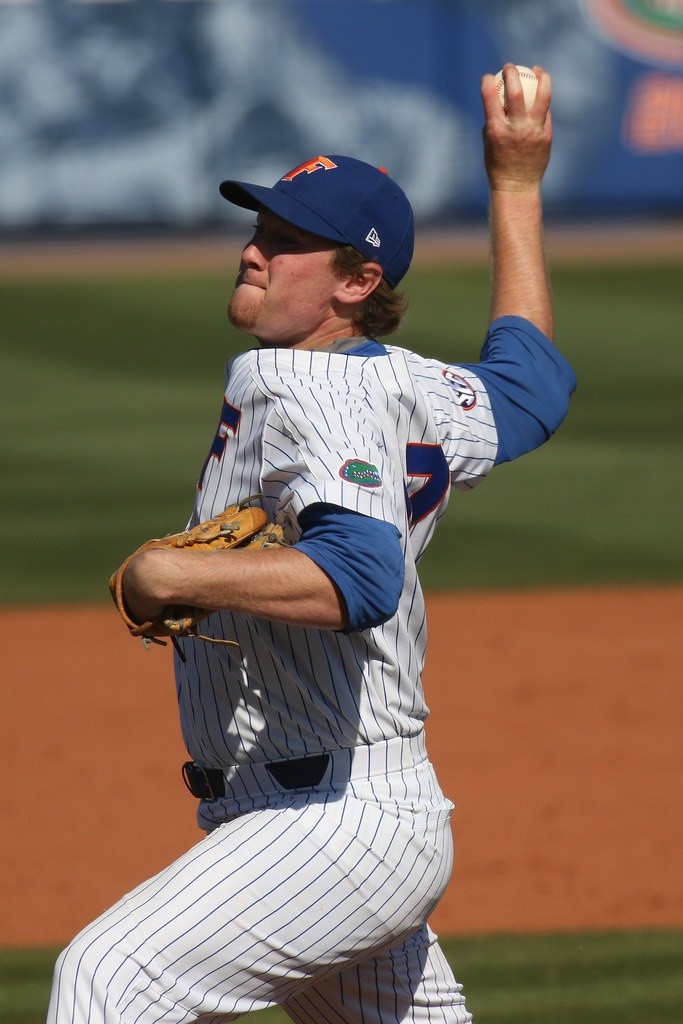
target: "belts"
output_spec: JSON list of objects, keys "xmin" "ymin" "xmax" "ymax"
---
[{"xmin": 179, "ymin": 754, "xmax": 330, "ymax": 804}]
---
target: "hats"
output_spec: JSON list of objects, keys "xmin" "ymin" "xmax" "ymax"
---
[{"xmin": 219, "ymin": 154, "xmax": 417, "ymax": 291}]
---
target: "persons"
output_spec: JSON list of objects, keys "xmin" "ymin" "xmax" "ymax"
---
[{"xmin": 48, "ymin": 62, "xmax": 578, "ymax": 1024}]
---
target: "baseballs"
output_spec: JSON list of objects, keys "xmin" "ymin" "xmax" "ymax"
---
[{"xmin": 495, "ymin": 62, "xmax": 539, "ymax": 110}]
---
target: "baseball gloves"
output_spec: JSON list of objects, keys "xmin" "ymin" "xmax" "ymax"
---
[{"xmin": 106, "ymin": 491, "xmax": 284, "ymax": 654}]
